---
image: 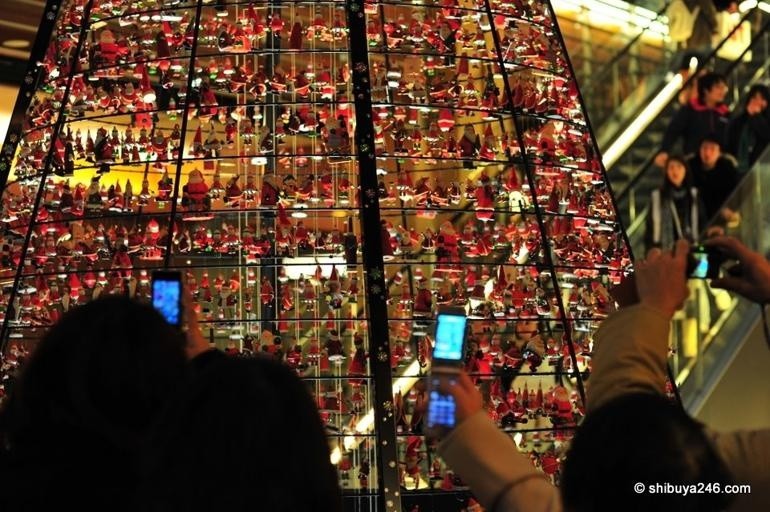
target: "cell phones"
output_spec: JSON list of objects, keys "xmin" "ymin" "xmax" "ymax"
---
[
  {"xmin": 150, "ymin": 270, "xmax": 183, "ymax": 327},
  {"xmin": 427, "ymin": 312, "xmax": 468, "ymax": 429}
]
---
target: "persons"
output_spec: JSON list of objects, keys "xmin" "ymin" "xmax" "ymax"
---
[
  {"xmin": 415, "ymin": 369, "xmax": 742, "ymax": 512},
  {"xmin": 128, "ymin": 348, "xmax": 344, "ymax": 512},
  {"xmin": 585, "ymin": 237, "xmax": 770, "ymax": 512},
  {"xmin": 644, "ymin": 0, "xmax": 770, "ymax": 261},
  {"xmin": 0, "ymin": 290, "xmax": 212, "ymax": 512}
]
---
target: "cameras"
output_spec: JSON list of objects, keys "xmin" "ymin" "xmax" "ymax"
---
[{"xmin": 686, "ymin": 247, "xmax": 722, "ymax": 280}]
